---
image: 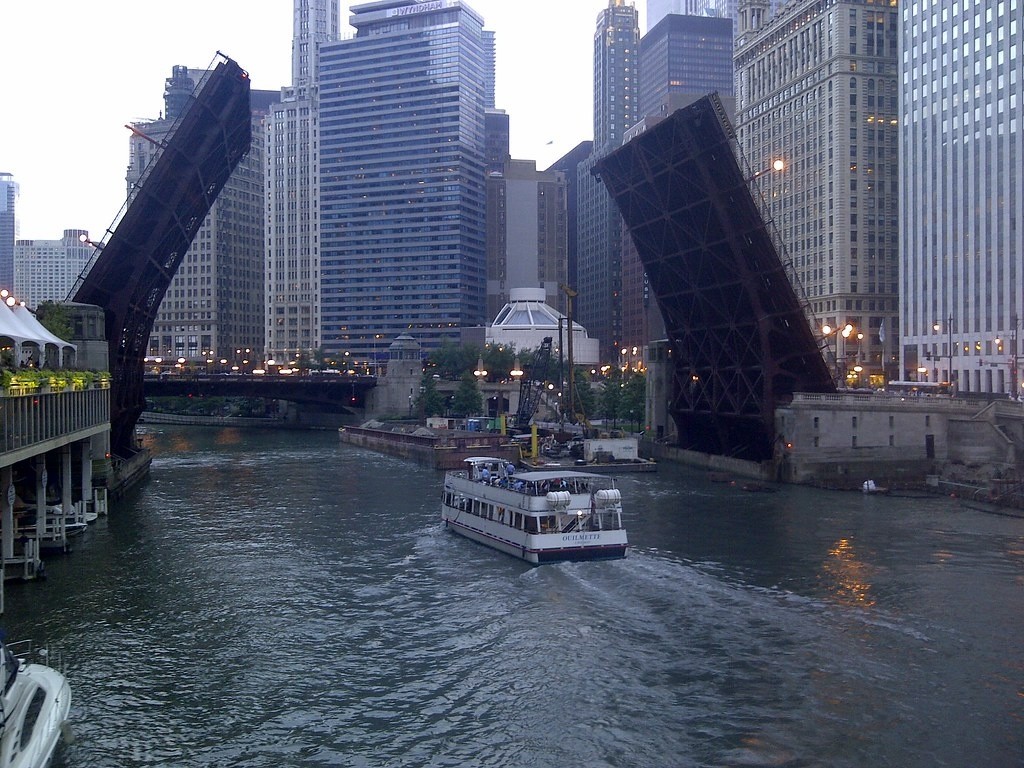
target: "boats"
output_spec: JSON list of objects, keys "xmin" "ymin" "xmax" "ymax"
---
[
  {"xmin": 11, "ymin": 502, "xmax": 98, "ymax": 552},
  {"xmin": 0, "ymin": 637, "xmax": 72, "ymax": 768},
  {"xmin": 441, "ymin": 457, "xmax": 630, "ymax": 565}
]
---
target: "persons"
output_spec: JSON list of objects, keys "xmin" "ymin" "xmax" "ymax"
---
[
  {"xmin": 482, "ymin": 466, "xmax": 490, "ymax": 486},
  {"xmin": 506, "ymin": 461, "xmax": 515, "ymax": 475},
  {"xmin": 489, "ymin": 473, "xmax": 590, "ymax": 496}
]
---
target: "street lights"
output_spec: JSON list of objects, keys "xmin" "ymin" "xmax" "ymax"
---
[
  {"xmin": 934, "ymin": 313, "xmax": 951, "ymax": 385},
  {"xmin": 994, "ymin": 330, "xmax": 1015, "ymax": 379}
]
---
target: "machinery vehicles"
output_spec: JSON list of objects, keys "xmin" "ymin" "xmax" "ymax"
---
[{"xmin": 505, "ymin": 335, "xmax": 553, "ymax": 435}]
